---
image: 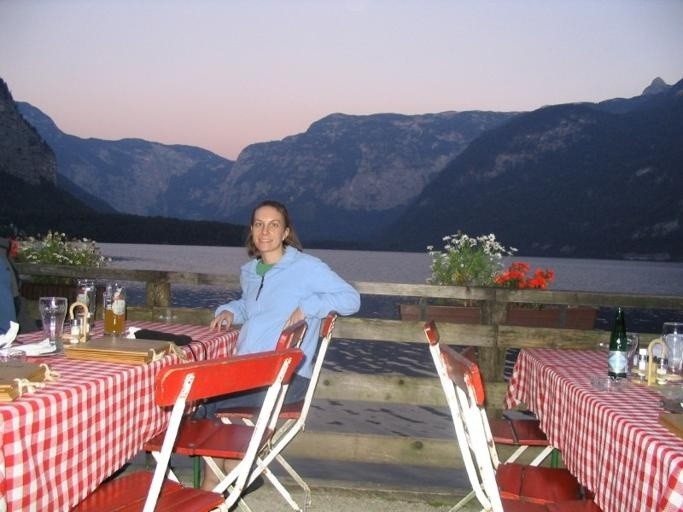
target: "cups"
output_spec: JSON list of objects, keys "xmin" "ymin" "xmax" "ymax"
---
[
  {"xmin": 0, "ymin": 347, "xmax": 26, "ymax": 385},
  {"xmin": 662, "ymin": 320, "xmax": 682, "ymax": 382},
  {"xmin": 624, "ymin": 332, "xmax": 638, "ymax": 377},
  {"xmin": 657, "ymin": 385, "xmax": 683, "ymax": 407},
  {"xmin": 76, "ymin": 280, "xmax": 98, "ymax": 334},
  {"xmin": 38, "ymin": 296, "xmax": 66, "ymax": 353}
]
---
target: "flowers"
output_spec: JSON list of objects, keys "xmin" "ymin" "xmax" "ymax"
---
[
  {"xmin": 424, "ymin": 229, "xmax": 554, "ymax": 289},
  {"xmin": 8, "ymin": 229, "xmax": 113, "ymax": 286}
]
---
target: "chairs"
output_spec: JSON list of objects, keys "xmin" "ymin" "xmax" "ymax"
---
[
  {"xmin": 143, "ymin": 319, "xmax": 309, "ymax": 512},
  {"xmin": 201, "ymin": 310, "xmax": 339, "ymax": 512},
  {"xmin": 438, "ymin": 341, "xmax": 603, "ymax": 512},
  {"xmin": 70, "ymin": 348, "xmax": 305, "ymax": 512},
  {"xmin": 421, "ymin": 316, "xmax": 559, "ymax": 468}
]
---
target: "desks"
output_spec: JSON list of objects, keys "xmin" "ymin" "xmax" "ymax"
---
[
  {"xmin": 1, "ymin": 318, "xmax": 241, "ymax": 512},
  {"xmin": 502, "ymin": 347, "xmax": 683, "ymax": 511}
]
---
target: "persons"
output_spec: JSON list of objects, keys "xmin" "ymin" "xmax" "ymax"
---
[{"xmin": 194, "ymin": 201, "xmax": 361, "ymax": 512}]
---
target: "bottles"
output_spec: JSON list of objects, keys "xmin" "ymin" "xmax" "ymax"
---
[
  {"xmin": 638, "ymin": 354, "xmax": 649, "ymax": 380},
  {"xmin": 103, "ymin": 280, "xmax": 126, "ymax": 335},
  {"xmin": 69, "ymin": 319, "xmax": 80, "ymax": 343},
  {"xmin": 656, "ymin": 356, "xmax": 669, "ymax": 383},
  {"xmin": 607, "ymin": 304, "xmax": 626, "ymax": 377}
]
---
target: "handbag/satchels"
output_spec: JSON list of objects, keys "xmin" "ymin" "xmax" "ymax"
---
[{"xmin": 134, "ymin": 329, "xmax": 191, "ymax": 346}]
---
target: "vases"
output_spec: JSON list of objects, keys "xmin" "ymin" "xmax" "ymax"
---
[
  {"xmin": 398, "ymin": 304, "xmax": 597, "ymax": 329},
  {"xmin": 20, "ymin": 279, "xmax": 85, "ymax": 320}
]
